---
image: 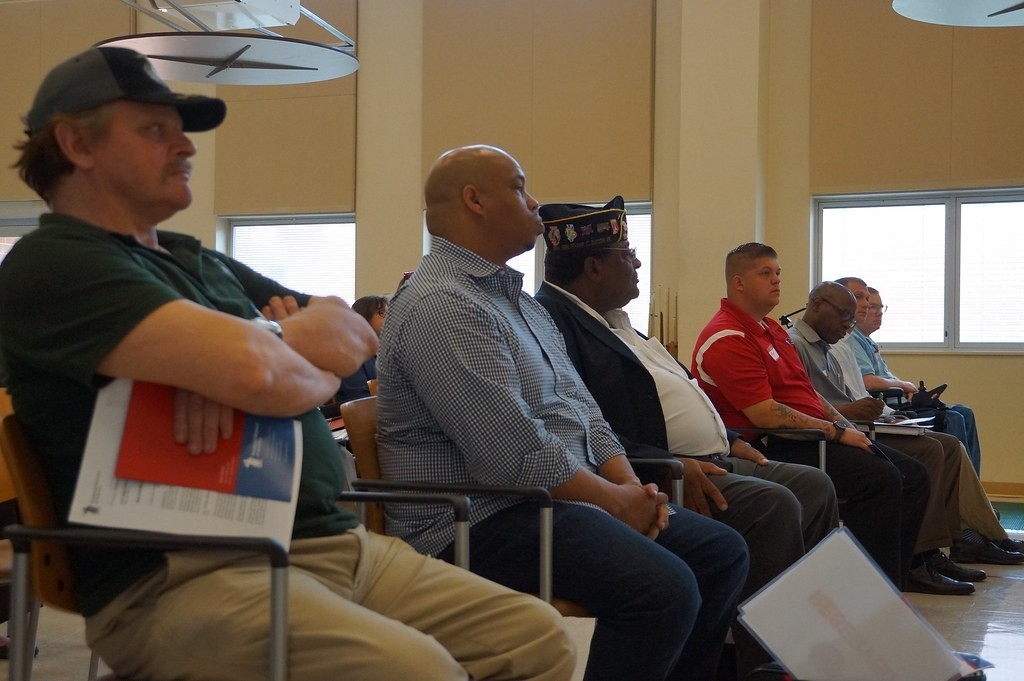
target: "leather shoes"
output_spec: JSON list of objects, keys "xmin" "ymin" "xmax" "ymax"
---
[
  {"xmin": 992, "ymin": 538, "xmax": 1024, "ymax": 553},
  {"xmin": 926, "ymin": 552, "xmax": 986, "ymax": 582},
  {"xmin": 902, "ymin": 562, "xmax": 975, "ymax": 595},
  {"xmin": 951, "ymin": 540, "xmax": 1024, "ymax": 563}
]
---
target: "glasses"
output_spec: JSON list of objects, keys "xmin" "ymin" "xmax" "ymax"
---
[
  {"xmin": 823, "ymin": 298, "xmax": 856, "ymax": 329},
  {"xmin": 375, "ymin": 309, "xmax": 388, "ymax": 318},
  {"xmin": 869, "ymin": 305, "xmax": 888, "ymax": 314},
  {"xmin": 592, "ymin": 248, "xmax": 636, "ymax": 261}
]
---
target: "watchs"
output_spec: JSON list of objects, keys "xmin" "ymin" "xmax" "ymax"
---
[{"xmin": 830, "ymin": 420, "xmax": 846, "ymax": 441}]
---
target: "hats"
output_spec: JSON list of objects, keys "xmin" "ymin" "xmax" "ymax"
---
[
  {"xmin": 538, "ymin": 196, "xmax": 628, "ymax": 252},
  {"xmin": 24, "ymin": 47, "xmax": 227, "ymax": 132}
]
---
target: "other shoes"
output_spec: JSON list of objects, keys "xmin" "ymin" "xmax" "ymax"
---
[{"xmin": 0, "ymin": 642, "xmax": 40, "ymax": 659}]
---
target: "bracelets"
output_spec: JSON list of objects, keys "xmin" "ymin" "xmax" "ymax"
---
[{"xmin": 270, "ymin": 319, "xmax": 285, "ymax": 337}]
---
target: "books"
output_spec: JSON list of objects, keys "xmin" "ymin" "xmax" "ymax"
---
[{"xmin": 857, "ymin": 424, "xmax": 926, "ymax": 436}]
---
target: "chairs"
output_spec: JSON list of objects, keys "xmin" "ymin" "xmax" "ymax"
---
[{"xmin": 0, "ymin": 381, "xmax": 904, "ymax": 680}]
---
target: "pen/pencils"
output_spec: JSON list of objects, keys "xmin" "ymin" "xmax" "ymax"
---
[{"xmin": 878, "ymin": 392, "xmax": 884, "ymax": 401}]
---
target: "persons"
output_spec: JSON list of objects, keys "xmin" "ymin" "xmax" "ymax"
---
[
  {"xmin": 0, "ymin": 46, "xmax": 580, "ymax": 681},
  {"xmin": 322, "ymin": 143, "xmax": 1024, "ymax": 681}
]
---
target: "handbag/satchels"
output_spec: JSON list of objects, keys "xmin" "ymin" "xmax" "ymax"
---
[{"xmin": 897, "ymin": 384, "xmax": 947, "ymax": 432}]
